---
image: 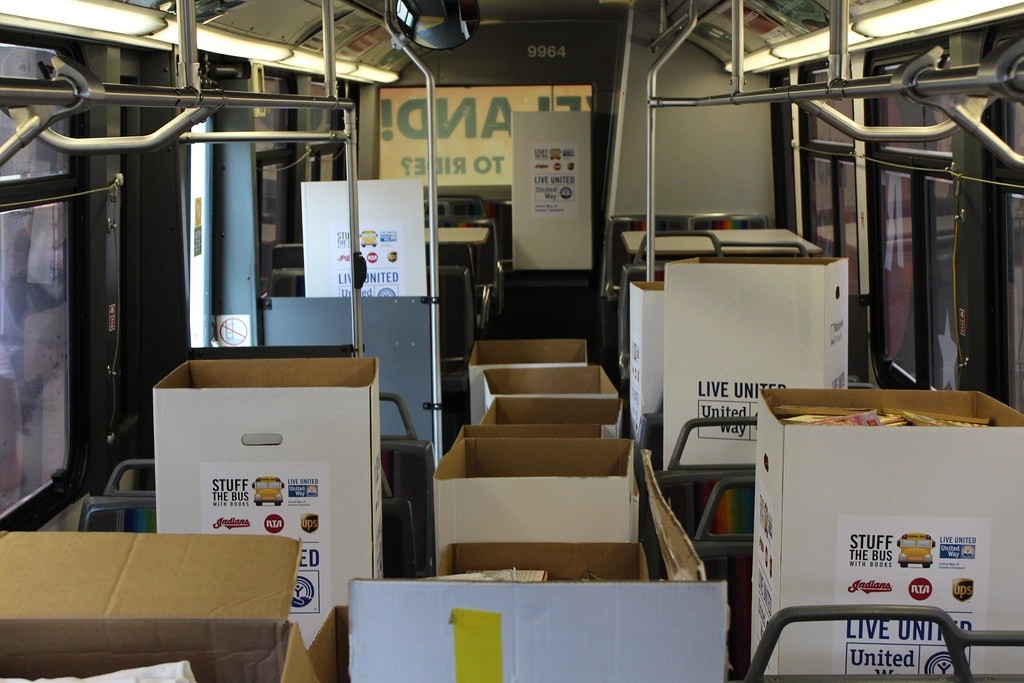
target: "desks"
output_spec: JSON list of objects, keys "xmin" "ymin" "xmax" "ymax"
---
[
  {"xmin": 426, "ymin": 228, "xmax": 490, "ymax": 245},
  {"xmin": 621, "ymin": 229, "xmax": 822, "ymax": 258}
]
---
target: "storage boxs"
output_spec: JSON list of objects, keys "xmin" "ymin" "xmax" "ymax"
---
[
  {"xmin": 450, "ymin": 424, "xmax": 603, "ymax": 438},
  {"xmin": 0, "ymin": 530, "xmax": 323, "ymax": 683},
  {"xmin": 508, "ymin": 108, "xmax": 594, "ymax": 272},
  {"xmin": 484, "ymin": 365, "xmax": 619, "ymax": 415},
  {"xmin": 433, "ymin": 439, "xmax": 640, "ymax": 575},
  {"xmin": 479, "ymin": 397, "xmax": 624, "ymax": 440},
  {"xmin": 438, "ymin": 543, "xmax": 649, "ymax": 582},
  {"xmin": 469, "ymin": 339, "xmax": 587, "ymax": 424},
  {"xmin": 752, "ymin": 389, "xmax": 1024, "ymax": 675},
  {"xmin": 630, "ymin": 257, "xmax": 849, "ymax": 470},
  {"xmin": 308, "ymin": 605, "xmax": 351, "ymax": 683},
  {"xmin": 153, "ymin": 358, "xmax": 381, "ymax": 649}
]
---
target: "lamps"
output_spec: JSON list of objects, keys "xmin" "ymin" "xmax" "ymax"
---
[
  {"xmin": 724, "ymin": 0, "xmax": 1024, "ymax": 73},
  {"xmin": 0, "ymin": 0, "xmax": 401, "ymax": 83}
]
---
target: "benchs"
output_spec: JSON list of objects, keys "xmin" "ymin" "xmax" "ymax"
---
[
  {"xmin": 638, "ymin": 390, "xmax": 664, "ymax": 469},
  {"xmin": 78, "ymin": 393, "xmax": 436, "ymax": 580},
  {"xmin": 606, "ymin": 216, "xmax": 768, "ymax": 300},
  {"xmin": 269, "ymin": 194, "xmax": 499, "ymax": 392},
  {"xmin": 658, "ymin": 416, "xmax": 1024, "ymax": 683}
]
